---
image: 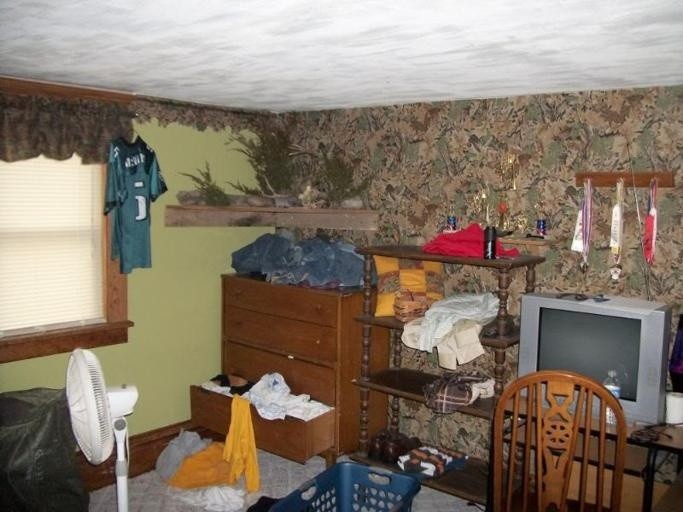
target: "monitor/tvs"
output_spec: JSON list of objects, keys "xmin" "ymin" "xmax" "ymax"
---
[{"xmin": 515, "ymin": 292, "xmax": 672, "ymax": 427}]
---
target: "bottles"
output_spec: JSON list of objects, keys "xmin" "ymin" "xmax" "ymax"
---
[
  {"xmin": 602, "ymin": 369, "xmax": 620, "ymax": 424},
  {"xmin": 368, "ymin": 437, "xmax": 401, "ymax": 464},
  {"xmin": 446, "ymin": 205, "xmax": 456, "ymax": 230},
  {"xmin": 483, "ymin": 226, "xmax": 496, "ymax": 259},
  {"xmin": 536, "ymin": 203, "xmax": 545, "ymax": 235}
]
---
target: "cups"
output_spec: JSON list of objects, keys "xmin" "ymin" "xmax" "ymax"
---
[{"xmin": 664, "ymin": 391, "xmax": 683, "ymax": 427}]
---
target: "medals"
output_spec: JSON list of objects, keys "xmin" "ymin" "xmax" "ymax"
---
[
  {"xmin": 579, "ymin": 261, "xmax": 589, "ymax": 273},
  {"xmin": 611, "ymin": 264, "xmax": 621, "ymax": 280}
]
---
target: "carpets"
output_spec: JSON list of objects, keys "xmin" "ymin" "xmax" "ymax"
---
[{"xmin": 84, "ymin": 439, "xmax": 486, "ymax": 509}]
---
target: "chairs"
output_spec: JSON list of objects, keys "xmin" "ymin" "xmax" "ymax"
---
[{"xmin": 486, "ymin": 368, "xmax": 627, "ymax": 511}]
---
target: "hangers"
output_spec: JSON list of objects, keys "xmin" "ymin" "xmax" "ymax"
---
[{"xmin": 119, "ymin": 113, "xmax": 141, "ymax": 142}]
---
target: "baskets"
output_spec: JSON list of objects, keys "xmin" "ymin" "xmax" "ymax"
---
[
  {"xmin": 270, "ymin": 460, "xmax": 421, "ymax": 511},
  {"xmin": 496, "ymin": 234, "xmax": 553, "ymax": 257},
  {"xmin": 393, "ymin": 295, "xmax": 428, "ymax": 323}
]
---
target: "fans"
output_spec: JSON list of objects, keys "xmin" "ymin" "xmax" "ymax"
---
[{"xmin": 61, "ymin": 346, "xmax": 143, "ymax": 512}]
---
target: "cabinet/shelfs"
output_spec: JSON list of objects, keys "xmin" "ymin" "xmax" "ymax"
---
[
  {"xmin": 489, "ymin": 379, "xmax": 670, "ymax": 510},
  {"xmin": 353, "ymin": 236, "xmax": 542, "ymax": 509},
  {"xmin": 186, "ymin": 271, "xmax": 390, "ymax": 471}
]
